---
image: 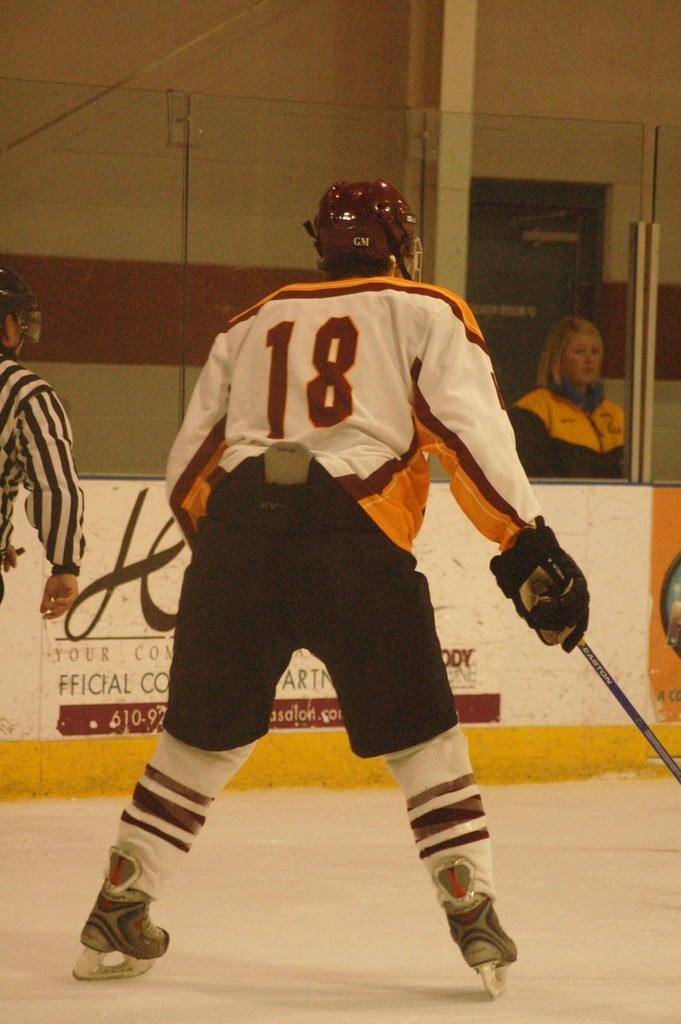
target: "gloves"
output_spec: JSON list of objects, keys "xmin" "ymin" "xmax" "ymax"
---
[{"xmin": 490, "ymin": 517, "xmax": 590, "ymax": 653}]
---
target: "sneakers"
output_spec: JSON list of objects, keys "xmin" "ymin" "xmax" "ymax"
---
[
  {"xmin": 72, "ymin": 846, "xmax": 169, "ymax": 980},
  {"xmin": 433, "ymin": 858, "xmax": 518, "ymax": 999}
]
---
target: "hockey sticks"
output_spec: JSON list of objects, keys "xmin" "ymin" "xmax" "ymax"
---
[{"xmin": 529, "ymin": 574, "xmax": 681, "ymax": 785}]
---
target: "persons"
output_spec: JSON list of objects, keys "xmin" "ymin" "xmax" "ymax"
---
[
  {"xmin": 508, "ymin": 313, "xmax": 626, "ymax": 478},
  {"xmin": 82, "ymin": 176, "xmax": 590, "ymax": 968},
  {"xmin": 0, "ymin": 270, "xmax": 86, "ymax": 618}
]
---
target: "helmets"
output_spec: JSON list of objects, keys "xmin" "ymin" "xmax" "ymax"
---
[
  {"xmin": 316, "ymin": 179, "xmax": 422, "ymax": 281},
  {"xmin": 0, "ymin": 267, "xmax": 36, "ymax": 322}
]
